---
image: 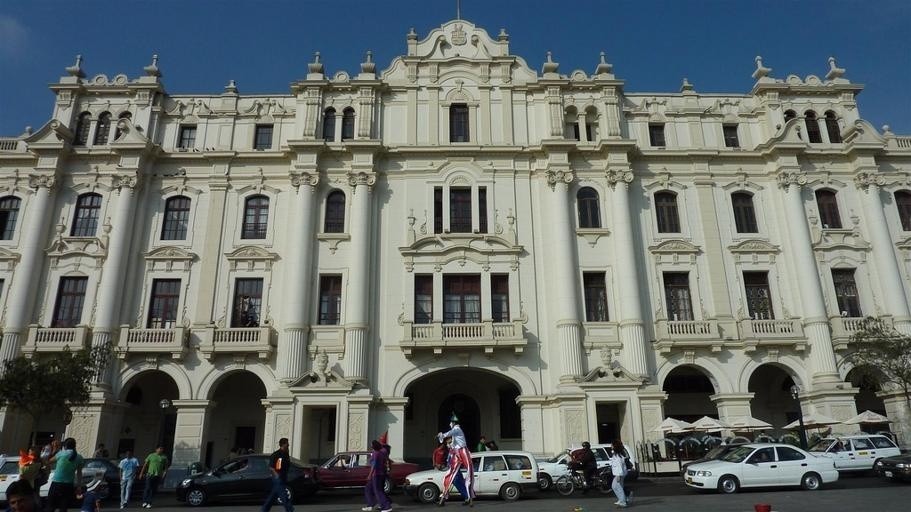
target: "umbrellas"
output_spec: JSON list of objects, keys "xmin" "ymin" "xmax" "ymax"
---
[{"xmin": 647, "ymin": 410, "xmax": 894, "ymax": 459}]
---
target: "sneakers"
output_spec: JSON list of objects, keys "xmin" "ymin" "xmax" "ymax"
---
[
  {"xmin": 143, "ymin": 502, "xmax": 151, "ymax": 509},
  {"xmin": 614, "ymin": 490, "xmax": 633, "ymax": 506},
  {"xmin": 362, "ymin": 500, "xmax": 392, "ymax": 512}
]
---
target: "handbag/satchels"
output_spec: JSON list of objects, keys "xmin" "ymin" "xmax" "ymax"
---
[
  {"xmin": 625, "ymin": 458, "xmax": 633, "ymax": 469},
  {"xmin": 35, "ymin": 472, "xmax": 47, "ymax": 487}
]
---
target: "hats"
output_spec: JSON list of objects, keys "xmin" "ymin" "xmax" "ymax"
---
[{"xmin": 85, "ymin": 479, "xmax": 101, "ymax": 491}]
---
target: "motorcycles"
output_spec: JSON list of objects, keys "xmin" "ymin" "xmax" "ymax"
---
[{"xmin": 556, "ymin": 447, "xmax": 621, "ymax": 495}]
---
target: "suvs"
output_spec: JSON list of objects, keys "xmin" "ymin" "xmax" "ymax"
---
[
  {"xmin": 810, "ymin": 434, "xmax": 902, "ymax": 477},
  {"xmin": 533, "ymin": 443, "xmax": 642, "ymax": 487},
  {"xmin": 401, "ymin": 450, "xmax": 540, "ymax": 501}
]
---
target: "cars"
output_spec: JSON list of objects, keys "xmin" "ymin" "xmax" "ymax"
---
[
  {"xmin": 680, "ymin": 441, "xmax": 747, "ymax": 480},
  {"xmin": 875, "ymin": 450, "xmax": 911, "ymax": 481},
  {"xmin": 176, "ymin": 452, "xmax": 320, "ymax": 506},
  {"xmin": 318, "ymin": 449, "xmax": 421, "ymax": 493},
  {"xmin": 0, "ymin": 449, "xmax": 167, "ymax": 507},
  {"xmin": 685, "ymin": 443, "xmax": 841, "ymax": 490}
]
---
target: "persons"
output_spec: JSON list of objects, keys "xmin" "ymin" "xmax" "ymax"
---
[
  {"xmin": 577, "ymin": 442, "xmax": 596, "ymax": 494},
  {"xmin": 228, "ymin": 448, "xmax": 255, "ymax": 462},
  {"xmin": 259, "ymin": 438, "xmax": 295, "ymax": 512},
  {"xmin": 6, "ymin": 438, "xmax": 168, "ymax": 512},
  {"xmin": 609, "ymin": 439, "xmax": 633, "ymax": 507},
  {"xmin": 361, "ymin": 415, "xmax": 498, "ymax": 512}
]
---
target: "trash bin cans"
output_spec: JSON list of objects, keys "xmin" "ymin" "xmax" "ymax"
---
[{"xmin": 190, "ymin": 462, "xmax": 202, "ymax": 475}]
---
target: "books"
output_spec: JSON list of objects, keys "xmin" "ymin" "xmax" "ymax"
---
[{"xmin": 276, "ymin": 458, "xmax": 282, "ymax": 470}]
